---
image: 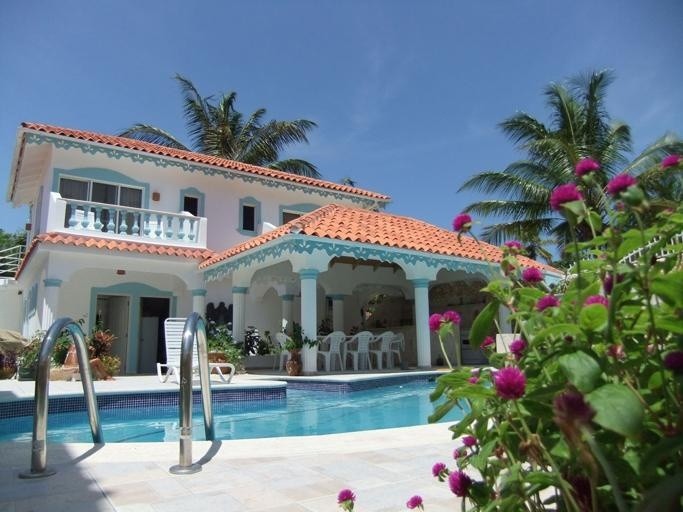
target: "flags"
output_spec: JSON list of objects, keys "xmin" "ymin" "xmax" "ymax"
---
[{"xmin": 210, "ymin": 323, "xmax": 246, "ymax": 375}]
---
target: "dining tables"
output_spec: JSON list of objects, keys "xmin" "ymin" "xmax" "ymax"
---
[{"xmin": 282, "ymin": 325, "xmax": 319, "ymax": 377}]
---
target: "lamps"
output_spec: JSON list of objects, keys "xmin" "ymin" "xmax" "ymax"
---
[
  {"xmin": 390, "ymin": 333, "xmax": 404, "ymax": 364},
  {"xmin": 318, "ymin": 331, "xmax": 346, "ymax": 372},
  {"xmin": 156, "ymin": 316, "xmax": 244, "ymax": 383},
  {"xmin": 273, "ymin": 331, "xmax": 297, "ymax": 373},
  {"xmin": 344, "ymin": 330, "xmax": 376, "ymax": 371},
  {"xmin": 373, "ymin": 330, "xmax": 395, "ymax": 370}
]
